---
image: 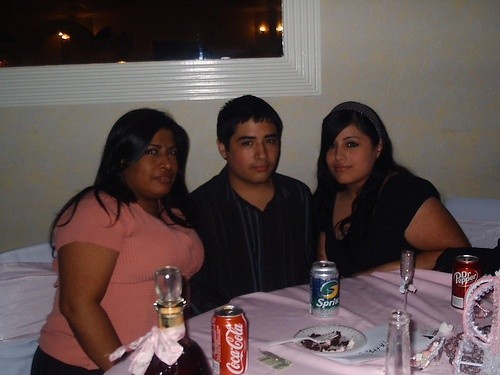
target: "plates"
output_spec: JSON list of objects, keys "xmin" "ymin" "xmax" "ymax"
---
[{"xmin": 293, "ymin": 324, "xmax": 367, "ymax": 356}]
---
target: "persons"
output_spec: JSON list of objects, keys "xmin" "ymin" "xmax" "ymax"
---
[
  {"xmin": 309, "ymin": 101, "xmax": 472, "ymax": 278},
  {"xmin": 183, "ymin": 94, "xmax": 314, "ymax": 317},
  {"xmin": 30, "ymin": 107, "xmax": 204, "ymax": 375}
]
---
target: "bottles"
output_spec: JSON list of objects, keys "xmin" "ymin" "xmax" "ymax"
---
[
  {"xmin": 383, "ymin": 310, "xmax": 411, "ymax": 375},
  {"xmin": 145, "ymin": 265, "xmax": 214, "ymax": 375}
]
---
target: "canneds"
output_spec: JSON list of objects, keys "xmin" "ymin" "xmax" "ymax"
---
[
  {"xmin": 210, "ymin": 305, "xmax": 250, "ymax": 375},
  {"xmin": 451, "ymin": 255, "xmax": 481, "ymax": 310},
  {"xmin": 309, "ymin": 260, "xmax": 340, "ymax": 317}
]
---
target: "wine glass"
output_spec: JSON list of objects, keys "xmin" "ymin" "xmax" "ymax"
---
[{"xmin": 395, "ymin": 250, "xmax": 414, "ymax": 317}]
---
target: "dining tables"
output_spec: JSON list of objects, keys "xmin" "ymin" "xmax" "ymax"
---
[{"xmin": 104, "ymin": 268, "xmax": 500, "ymax": 375}]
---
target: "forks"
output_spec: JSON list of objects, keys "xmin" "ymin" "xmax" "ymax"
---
[{"xmin": 262, "ymin": 333, "xmax": 338, "ymax": 346}]
